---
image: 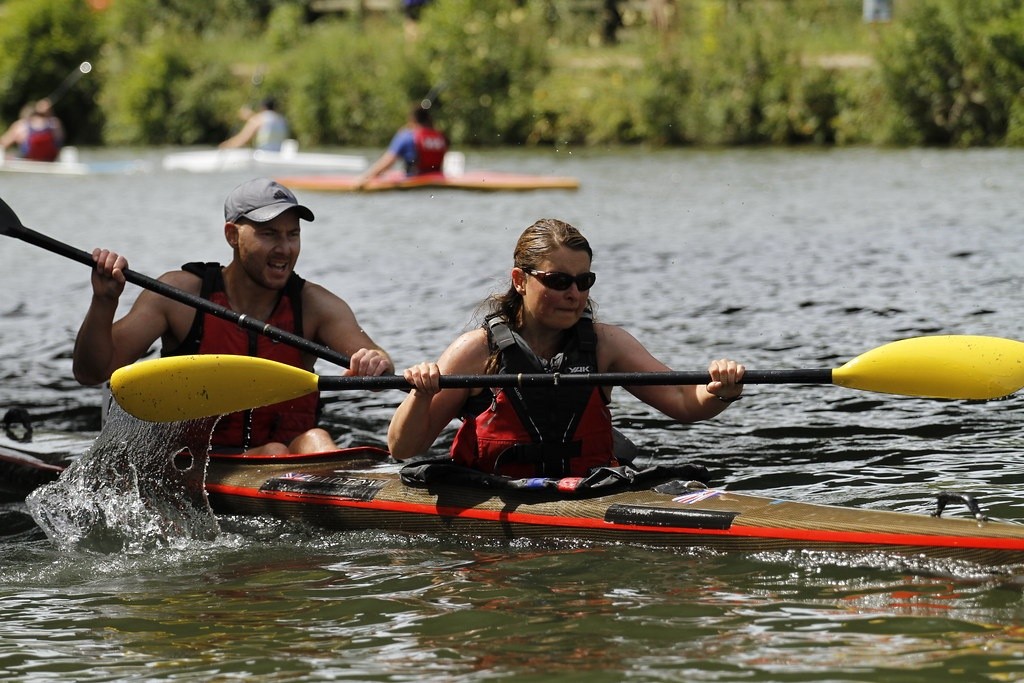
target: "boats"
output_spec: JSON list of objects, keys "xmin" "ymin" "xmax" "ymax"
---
[
  {"xmin": 0, "ymin": 393, "xmax": 1023, "ymax": 575},
  {"xmin": 166, "ymin": 144, "xmax": 370, "ymax": 178},
  {"xmin": 2, "ymin": 148, "xmax": 88, "ymax": 177},
  {"xmin": 275, "ymin": 170, "xmax": 580, "ymax": 195}
]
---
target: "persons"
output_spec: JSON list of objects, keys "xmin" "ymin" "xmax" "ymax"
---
[
  {"xmin": 387, "ymin": 220, "xmax": 744, "ymax": 477},
  {"xmin": 352, "ymin": 106, "xmax": 447, "ymax": 185},
  {"xmin": 220, "ymin": 96, "xmax": 291, "ymax": 153},
  {"xmin": 0, "ymin": 101, "xmax": 63, "ymax": 162},
  {"xmin": 73, "ymin": 178, "xmax": 394, "ymax": 455}
]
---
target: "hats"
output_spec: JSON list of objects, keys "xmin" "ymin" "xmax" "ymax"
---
[{"xmin": 223, "ymin": 176, "xmax": 315, "ymax": 225}]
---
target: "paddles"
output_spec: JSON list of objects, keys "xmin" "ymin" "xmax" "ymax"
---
[
  {"xmin": 106, "ymin": 336, "xmax": 1024, "ymax": 426},
  {"xmin": 0, "ymin": 198, "xmax": 421, "ymax": 398}
]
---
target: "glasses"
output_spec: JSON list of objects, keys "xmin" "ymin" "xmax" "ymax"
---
[{"xmin": 522, "ymin": 267, "xmax": 596, "ymax": 292}]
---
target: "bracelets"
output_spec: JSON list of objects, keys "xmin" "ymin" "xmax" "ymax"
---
[{"xmin": 716, "ymin": 396, "xmax": 743, "ymax": 402}]
